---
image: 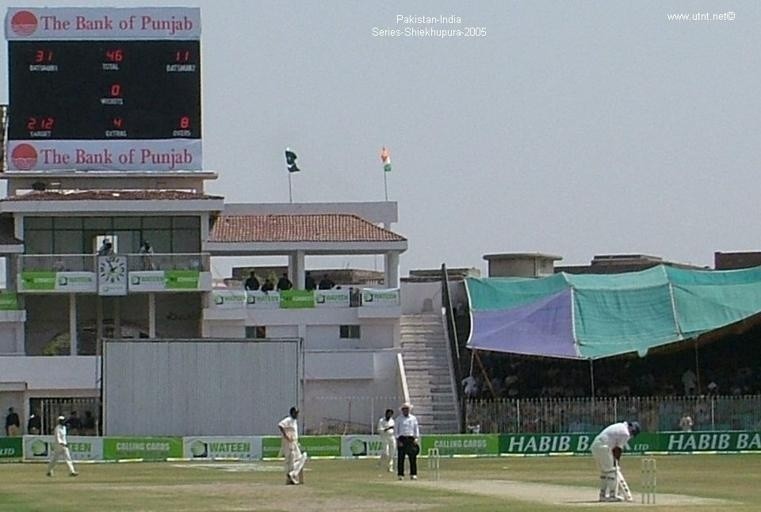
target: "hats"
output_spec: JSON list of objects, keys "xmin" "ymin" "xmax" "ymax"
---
[
  {"xmin": 398, "ymin": 402, "xmax": 413, "ymax": 412},
  {"xmin": 631, "ymin": 422, "xmax": 640, "ymax": 439}
]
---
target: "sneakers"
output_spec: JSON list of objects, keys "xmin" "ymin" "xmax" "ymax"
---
[
  {"xmin": 410, "ymin": 475, "xmax": 416, "ymax": 480},
  {"xmin": 599, "ymin": 497, "xmax": 608, "ymax": 501},
  {"xmin": 608, "ymin": 496, "xmax": 621, "ymax": 502},
  {"xmin": 287, "ymin": 471, "xmax": 296, "ymax": 485},
  {"xmin": 397, "ymin": 475, "xmax": 402, "ymax": 480}
]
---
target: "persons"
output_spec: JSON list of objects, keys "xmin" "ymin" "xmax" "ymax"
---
[
  {"xmin": 375, "ymin": 407, "xmax": 398, "ymax": 478},
  {"xmin": 47, "ymin": 413, "xmax": 82, "ymax": 477},
  {"xmin": 243, "ymin": 269, "xmax": 337, "ymax": 291},
  {"xmin": 591, "ymin": 420, "xmax": 642, "ymax": 501},
  {"xmin": 391, "ymin": 403, "xmax": 422, "ymax": 479},
  {"xmin": 5, "ymin": 405, "xmax": 97, "ymax": 436},
  {"xmin": 453, "ymin": 301, "xmax": 760, "ymax": 433},
  {"xmin": 140, "ymin": 239, "xmax": 156, "ymax": 269},
  {"xmin": 97, "ymin": 238, "xmax": 115, "ymax": 253},
  {"xmin": 279, "ymin": 408, "xmax": 308, "ymax": 486}
]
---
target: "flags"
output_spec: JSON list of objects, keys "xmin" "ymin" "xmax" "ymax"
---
[
  {"xmin": 380, "ymin": 145, "xmax": 391, "ymax": 170},
  {"xmin": 285, "ymin": 150, "xmax": 299, "ymax": 172}
]
